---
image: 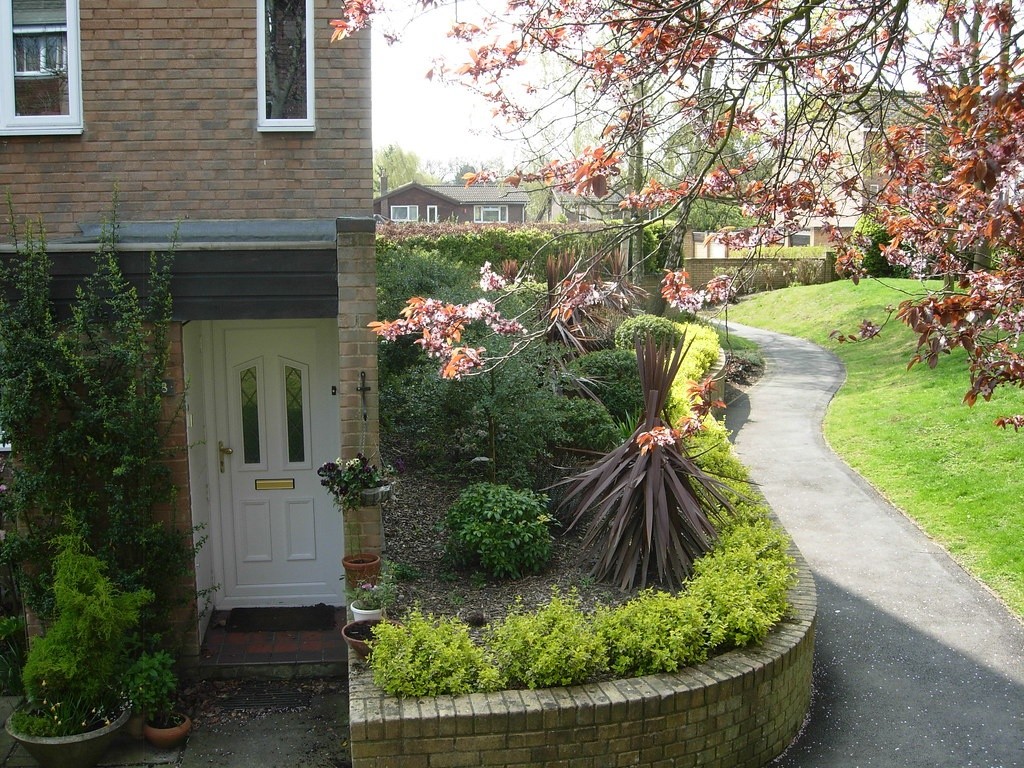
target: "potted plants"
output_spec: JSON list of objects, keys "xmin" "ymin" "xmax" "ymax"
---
[
  {"xmin": 130, "ymin": 650, "xmax": 191, "ymax": 749},
  {"xmin": 341, "ymin": 517, "xmax": 381, "ymax": 591},
  {"xmin": 3, "ymin": 500, "xmax": 156, "ymax": 768}
]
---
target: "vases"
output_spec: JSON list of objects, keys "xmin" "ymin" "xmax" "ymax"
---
[
  {"xmin": 341, "ymin": 620, "xmax": 405, "ymax": 659},
  {"xmin": 354, "ymin": 485, "xmax": 390, "ymax": 507},
  {"xmin": 350, "ymin": 599, "xmax": 385, "ymax": 621}
]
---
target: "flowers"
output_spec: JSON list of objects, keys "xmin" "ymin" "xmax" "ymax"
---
[
  {"xmin": 339, "ymin": 570, "xmax": 396, "ymax": 610},
  {"xmin": 317, "ymin": 455, "xmax": 394, "ymax": 512}
]
